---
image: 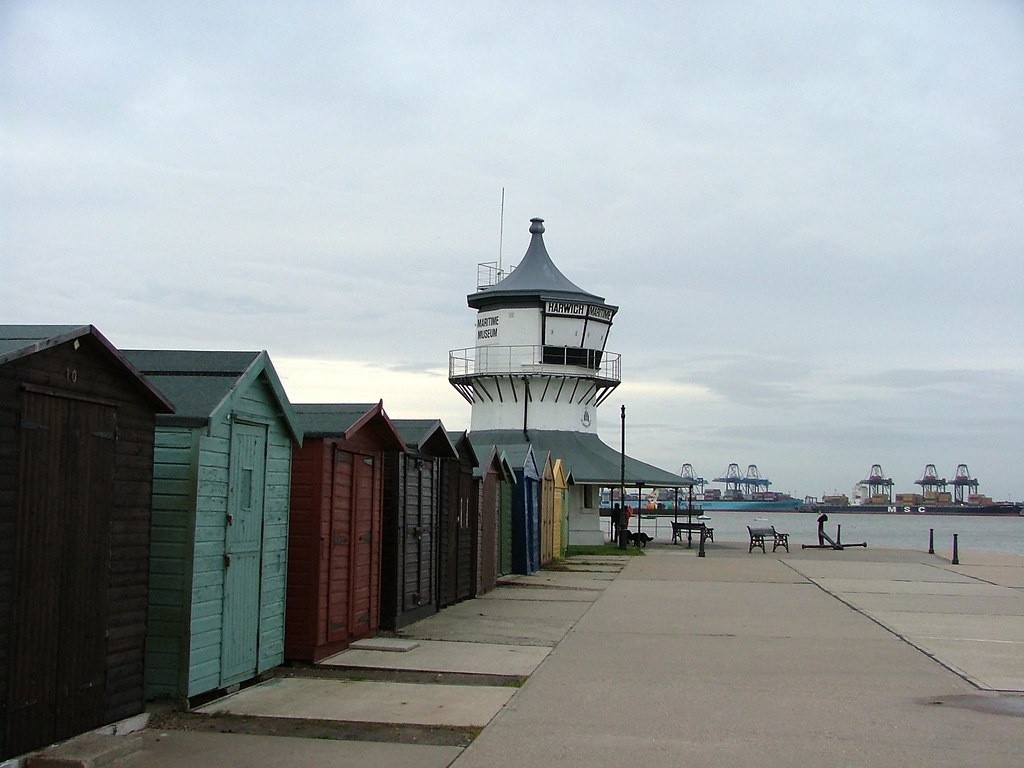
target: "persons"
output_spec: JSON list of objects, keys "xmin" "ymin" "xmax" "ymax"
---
[
  {"xmin": 624, "ymin": 506, "xmax": 631, "ymax": 528},
  {"xmin": 609, "ymin": 503, "xmax": 634, "ymax": 543}
]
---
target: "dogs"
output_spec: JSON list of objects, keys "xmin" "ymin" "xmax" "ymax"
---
[{"xmin": 628, "ymin": 533, "xmax": 654, "ymax": 547}]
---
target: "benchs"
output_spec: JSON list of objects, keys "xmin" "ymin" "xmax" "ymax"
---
[
  {"xmin": 747, "ymin": 526, "xmax": 790, "ymax": 554},
  {"xmin": 671, "ymin": 521, "xmax": 714, "ymax": 543}
]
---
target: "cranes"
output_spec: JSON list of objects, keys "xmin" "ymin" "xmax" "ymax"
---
[
  {"xmin": 679, "ymin": 463, "xmax": 708, "ymax": 501},
  {"xmin": 712, "ymin": 463, "xmax": 773, "ymax": 502},
  {"xmin": 947, "ymin": 464, "xmax": 980, "ymax": 505},
  {"xmin": 914, "ymin": 464, "xmax": 947, "ymax": 502},
  {"xmin": 859, "ymin": 464, "xmax": 895, "ymax": 505}
]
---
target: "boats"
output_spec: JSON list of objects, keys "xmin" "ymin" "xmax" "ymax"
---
[
  {"xmin": 697, "ymin": 515, "xmax": 711, "ymax": 519},
  {"xmin": 754, "ymin": 516, "xmax": 769, "ymax": 521}
]
